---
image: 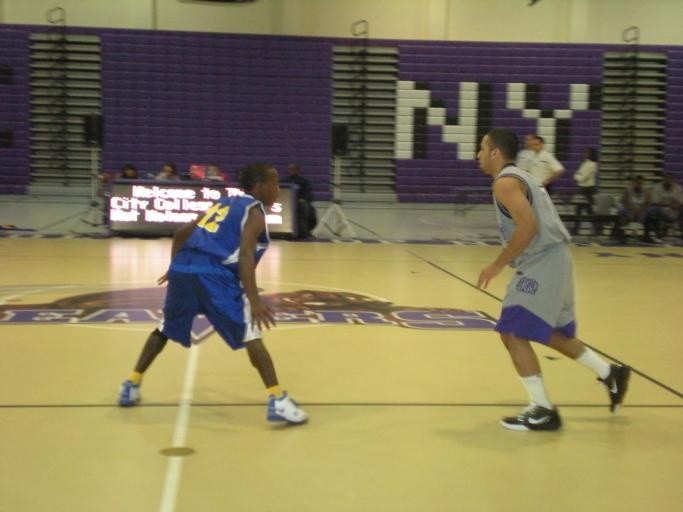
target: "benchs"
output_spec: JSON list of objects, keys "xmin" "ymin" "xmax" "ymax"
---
[{"xmin": 560, "ymin": 214, "xmax": 682, "ymax": 238}]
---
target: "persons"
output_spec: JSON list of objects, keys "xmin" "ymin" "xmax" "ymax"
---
[
  {"xmin": 516, "ymin": 132, "xmax": 534, "ymax": 171},
  {"xmin": 651, "ymin": 172, "xmax": 683, "ymax": 240},
  {"xmin": 153, "ymin": 163, "xmax": 180, "ymax": 181},
  {"xmin": 525, "ymin": 136, "xmax": 567, "ymax": 197},
  {"xmin": 281, "ymin": 162, "xmax": 318, "ymax": 238},
  {"xmin": 474, "ymin": 125, "xmax": 633, "ymax": 433},
  {"xmin": 113, "ymin": 164, "xmax": 142, "ymax": 179},
  {"xmin": 618, "ymin": 175, "xmax": 663, "ymax": 242},
  {"xmin": 570, "ymin": 146, "xmax": 603, "ymax": 237},
  {"xmin": 116, "ymin": 161, "xmax": 308, "ymax": 424}
]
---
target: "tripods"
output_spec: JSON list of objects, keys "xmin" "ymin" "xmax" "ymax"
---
[
  {"xmin": 312, "ymin": 154, "xmax": 355, "ymax": 238},
  {"xmin": 68, "ymin": 146, "xmax": 106, "ymax": 238}
]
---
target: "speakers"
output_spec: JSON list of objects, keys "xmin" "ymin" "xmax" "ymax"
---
[
  {"xmin": 331, "ymin": 124, "xmax": 347, "ymax": 155},
  {"xmin": 83, "ymin": 114, "xmax": 103, "ymax": 147}
]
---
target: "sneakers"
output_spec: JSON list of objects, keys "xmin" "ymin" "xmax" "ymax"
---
[
  {"xmin": 266, "ymin": 390, "xmax": 307, "ymax": 425},
  {"xmin": 119, "ymin": 381, "xmax": 141, "ymax": 405},
  {"xmin": 568, "ymin": 230, "xmax": 683, "ymax": 246},
  {"xmin": 596, "ymin": 364, "xmax": 629, "ymax": 413},
  {"xmin": 498, "ymin": 402, "xmax": 560, "ymax": 431}
]
